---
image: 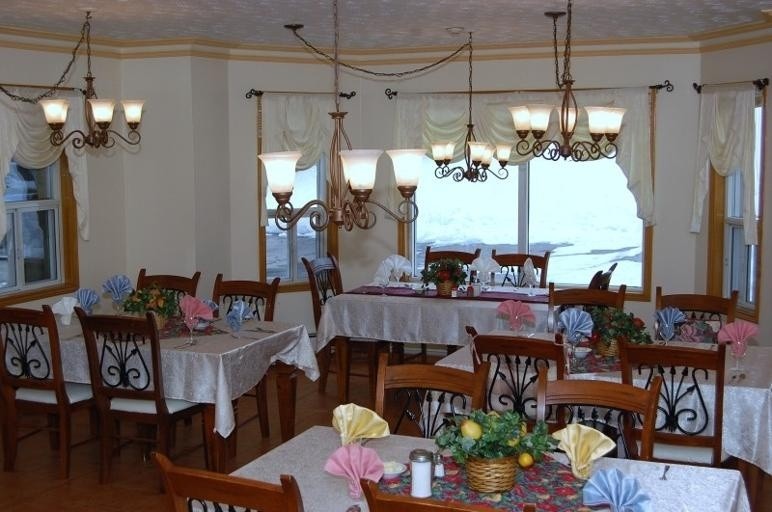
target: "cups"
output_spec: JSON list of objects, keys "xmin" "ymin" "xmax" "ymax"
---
[
  {"xmin": 659, "ymin": 324, "xmax": 674, "ymax": 345},
  {"xmin": 185, "ymin": 313, "xmax": 199, "ymax": 344},
  {"xmin": 394, "ymin": 268, "xmax": 403, "ymax": 284},
  {"xmin": 729, "ymin": 341, "xmax": 747, "ymax": 373},
  {"xmin": 478, "ymin": 272, "xmax": 490, "ymax": 292},
  {"xmin": 526, "ymin": 274, "xmax": 536, "ymax": 297},
  {"xmin": 378, "ymin": 278, "xmax": 390, "ymax": 297}
]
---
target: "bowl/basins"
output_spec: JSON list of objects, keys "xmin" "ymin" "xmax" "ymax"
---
[
  {"xmin": 574, "ymin": 346, "xmax": 592, "ymax": 359},
  {"xmin": 382, "ymin": 461, "xmax": 408, "ymax": 479}
]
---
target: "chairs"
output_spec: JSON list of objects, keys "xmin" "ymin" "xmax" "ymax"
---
[
  {"xmin": 74, "ymin": 306, "xmax": 215, "ymax": 494},
  {"xmin": 360, "ymin": 478, "xmax": 537, "ymax": 512},
  {"xmin": 547, "ymin": 283, "xmax": 627, "ymax": 333},
  {"xmin": 213, "ymin": 273, "xmax": 281, "ymax": 457},
  {"xmin": 0, "ymin": 304, "xmax": 99, "ymax": 476},
  {"xmin": 466, "ymin": 325, "xmax": 566, "ymax": 433},
  {"xmin": 421, "ymin": 246, "xmax": 481, "ymax": 362},
  {"xmin": 491, "ymin": 249, "xmax": 551, "ymax": 289},
  {"xmin": 583, "ymin": 263, "xmax": 618, "ymax": 313},
  {"xmin": 302, "ymin": 250, "xmax": 404, "ymax": 403},
  {"xmin": 655, "ymin": 285, "xmax": 740, "ymax": 345},
  {"xmin": 619, "ymin": 336, "xmax": 727, "ymax": 466},
  {"xmin": 375, "ymin": 354, "xmax": 491, "ymax": 439},
  {"xmin": 536, "ymin": 365, "xmax": 662, "ymax": 462},
  {"xmin": 150, "ymin": 451, "xmax": 305, "ymax": 512},
  {"xmin": 137, "ymin": 268, "xmax": 201, "ymax": 298}
]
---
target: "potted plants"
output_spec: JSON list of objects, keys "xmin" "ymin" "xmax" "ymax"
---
[
  {"xmin": 435, "ymin": 409, "xmax": 549, "ymax": 494},
  {"xmin": 419, "ymin": 258, "xmax": 467, "ymax": 297},
  {"xmin": 591, "ymin": 304, "xmax": 654, "ymax": 357},
  {"xmin": 122, "ymin": 282, "xmax": 178, "ymax": 330}
]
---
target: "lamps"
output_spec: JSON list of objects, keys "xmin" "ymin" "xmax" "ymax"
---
[
  {"xmin": 429, "ymin": 29, "xmax": 513, "ymax": 183},
  {"xmin": 507, "ymin": 11, "xmax": 627, "ymax": 160},
  {"xmin": 257, "ymin": 0, "xmax": 428, "ymax": 232},
  {"xmin": 41, "ymin": 7, "xmax": 146, "ymax": 149}
]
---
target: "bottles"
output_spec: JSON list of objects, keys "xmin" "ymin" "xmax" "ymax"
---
[
  {"xmin": 477, "ymin": 279, "xmax": 482, "ymax": 294},
  {"xmin": 529, "ymin": 284, "xmax": 533, "ymax": 296},
  {"xmin": 435, "ymin": 455, "xmax": 445, "ymax": 477},
  {"xmin": 452, "ymin": 288, "xmax": 458, "ymax": 297},
  {"xmin": 409, "ymin": 448, "xmax": 434, "ymax": 499},
  {"xmin": 533, "ymin": 285, "xmax": 535, "ymax": 296}
]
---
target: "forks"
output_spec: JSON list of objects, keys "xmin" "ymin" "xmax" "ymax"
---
[{"xmin": 660, "ymin": 465, "xmax": 670, "ymax": 481}]
